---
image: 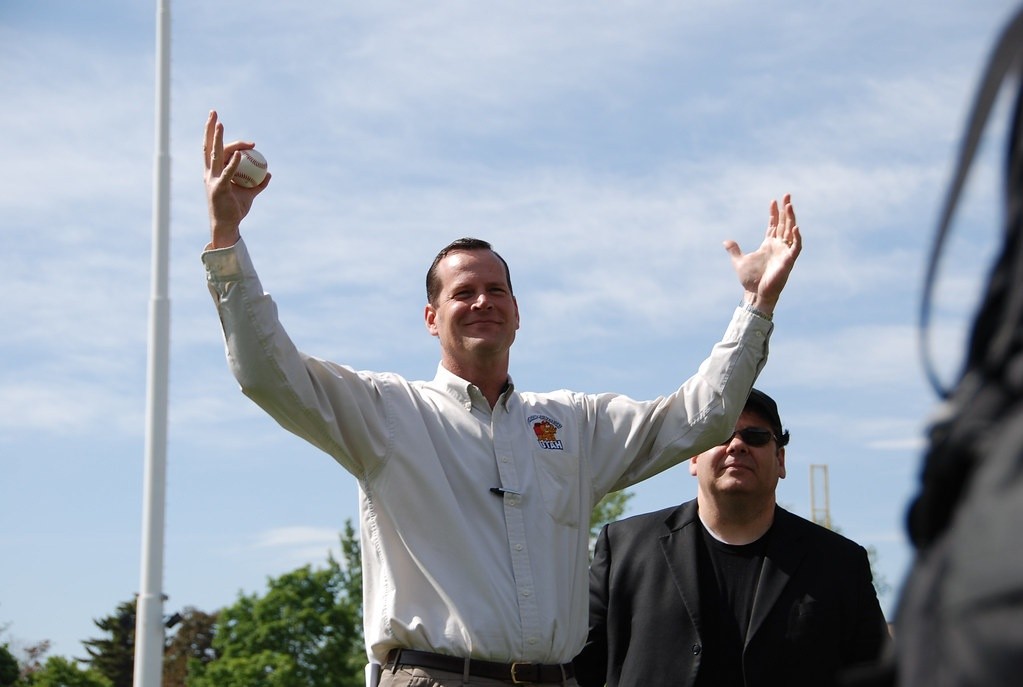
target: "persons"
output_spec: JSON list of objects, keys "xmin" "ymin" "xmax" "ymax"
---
[
  {"xmin": 198, "ymin": 110, "xmax": 803, "ymax": 687},
  {"xmin": 882, "ymin": 1, "xmax": 1023, "ymax": 686},
  {"xmin": 575, "ymin": 387, "xmax": 903, "ymax": 687}
]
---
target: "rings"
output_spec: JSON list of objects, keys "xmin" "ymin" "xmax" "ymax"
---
[{"xmin": 783, "ymin": 239, "xmax": 793, "ymax": 245}]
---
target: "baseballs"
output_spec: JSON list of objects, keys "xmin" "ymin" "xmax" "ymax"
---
[{"xmin": 227, "ymin": 148, "xmax": 268, "ymax": 189}]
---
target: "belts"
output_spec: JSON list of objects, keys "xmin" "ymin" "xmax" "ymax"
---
[{"xmin": 385, "ymin": 648, "xmax": 576, "ymax": 685}]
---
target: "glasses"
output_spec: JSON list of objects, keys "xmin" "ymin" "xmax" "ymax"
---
[{"xmin": 721, "ymin": 426, "xmax": 780, "ymax": 448}]
